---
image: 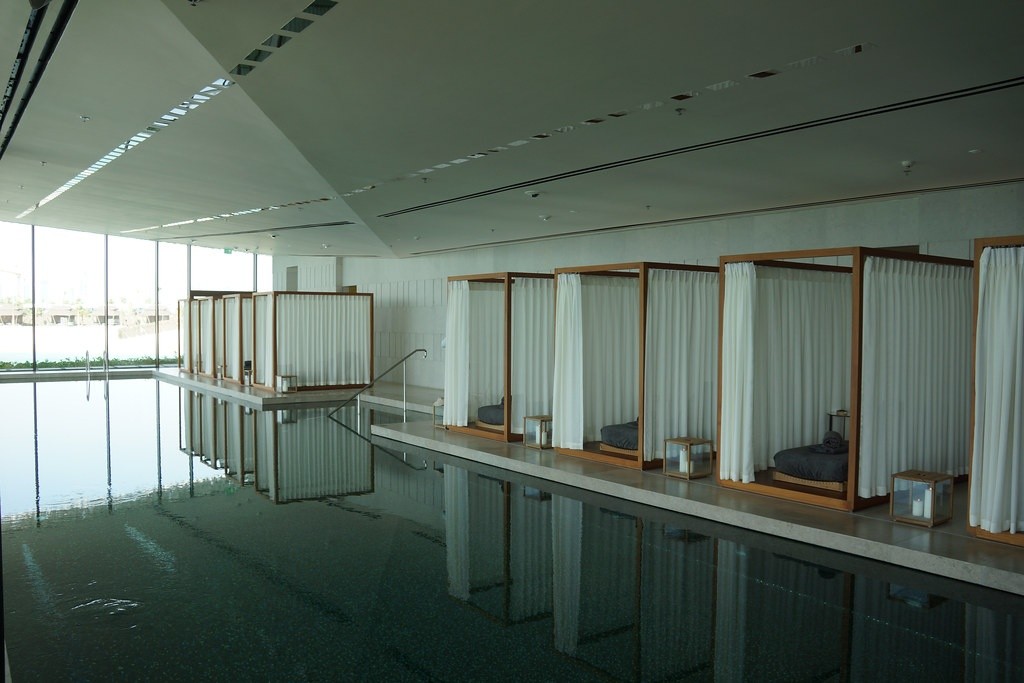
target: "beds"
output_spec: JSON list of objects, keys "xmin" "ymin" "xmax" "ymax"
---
[
  {"xmin": 474, "ymin": 397, "xmax": 505, "ymax": 431},
  {"xmin": 771, "ymin": 441, "xmax": 851, "ymax": 490},
  {"xmin": 599, "ymin": 417, "xmax": 640, "ymax": 455}
]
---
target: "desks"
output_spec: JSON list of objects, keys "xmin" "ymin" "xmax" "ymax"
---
[{"xmin": 827, "ymin": 413, "xmax": 850, "ymax": 440}]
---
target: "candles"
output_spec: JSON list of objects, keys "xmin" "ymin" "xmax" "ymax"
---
[
  {"xmin": 679, "ymin": 448, "xmax": 694, "ymax": 473},
  {"xmin": 542, "ymin": 430, "xmax": 547, "ymax": 445},
  {"xmin": 536, "ymin": 424, "xmax": 542, "ymax": 445},
  {"xmin": 912, "ymin": 498, "xmax": 924, "ymax": 516},
  {"xmin": 923, "ymin": 487, "xmax": 933, "ymax": 518}
]
